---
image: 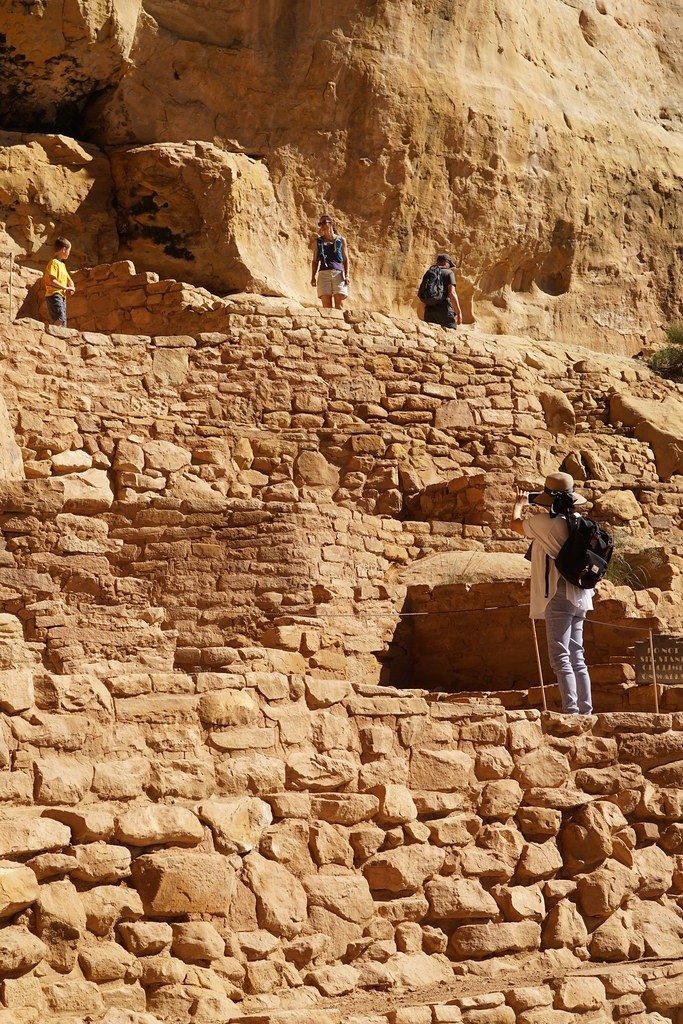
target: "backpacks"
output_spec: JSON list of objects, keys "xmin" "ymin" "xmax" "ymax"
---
[
  {"xmin": 417, "ymin": 265, "xmax": 444, "ymax": 306},
  {"xmin": 555, "ymin": 516, "xmax": 614, "ymax": 589}
]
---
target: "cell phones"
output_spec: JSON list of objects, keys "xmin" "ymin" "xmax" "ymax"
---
[{"xmin": 528, "ymin": 493, "xmax": 542, "ymax": 503}]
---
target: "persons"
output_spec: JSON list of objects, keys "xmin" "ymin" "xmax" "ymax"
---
[
  {"xmin": 45, "ymin": 237, "xmax": 75, "ymax": 327},
  {"xmin": 311, "ymin": 215, "xmax": 350, "ymax": 310},
  {"xmin": 511, "ymin": 472, "xmax": 595, "ymax": 715},
  {"xmin": 424, "ymin": 254, "xmax": 462, "ymax": 330}
]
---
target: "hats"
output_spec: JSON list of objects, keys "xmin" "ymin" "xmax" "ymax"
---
[
  {"xmin": 533, "ymin": 472, "xmax": 587, "ymax": 506},
  {"xmin": 437, "ymin": 253, "xmax": 456, "ymax": 267}
]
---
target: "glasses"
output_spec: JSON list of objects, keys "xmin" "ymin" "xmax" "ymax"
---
[{"xmin": 318, "ymin": 220, "xmax": 331, "ymax": 226}]
